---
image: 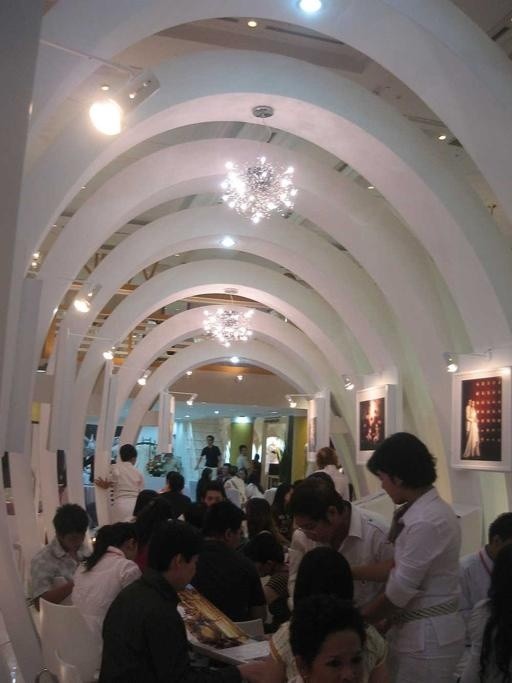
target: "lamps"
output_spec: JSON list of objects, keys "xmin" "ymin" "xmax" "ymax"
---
[
  {"xmin": 342, "ymin": 374, "xmax": 380, "ymax": 390},
  {"xmin": 27, "ymin": 275, "xmax": 102, "ymax": 313},
  {"xmin": 285, "ymin": 393, "xmax": 319, "ymax": 408},
  {"xmin": 218, "ymin": 106, "xmax": 298, "ymax": 225},
  {"xmin": 69, "ymin": 333, "xmax": 116, "ymax": 360},
  {"xmin": 112, "ymin": 365, "xmax": 151, "ymax": 386},
  {"xmin": 168, "ymin": 391, "xmax": 199, "ymax": 406},
  {"xmin": 39, "ymin": 38, "xmax": 161, "ymax": 137},
  {"xmin": 443, "ymin": 348, "xmax": 493, "ymax": 373},
  {"xmin": 202, "ymin": 288, "xmax": 256, "ymax": 348}
]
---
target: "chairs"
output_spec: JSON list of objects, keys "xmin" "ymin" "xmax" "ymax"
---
[
  {"xmin": 37, "ymin": 597, "xmax": 99, "ymax": 679},
  {"xmin": 235, "ymin": 620, "xmax": 267, "ymax": 641},
  {"xmin": 53, "ymin": 649, "xmax": 85, "ymax": 683}
]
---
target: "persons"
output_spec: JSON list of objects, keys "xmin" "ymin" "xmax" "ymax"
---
[
  {"xmin": 24, "ymin": 434, "xmax": 512, "ymax": 683},
  {"xmin": 462, "ymin": 399, "xmax": 480, "ymax": 457}
]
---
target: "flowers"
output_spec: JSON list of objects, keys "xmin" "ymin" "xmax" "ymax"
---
[{"xmin": 145, "ymin": 453, "xmax": 184, "ymax": 478}]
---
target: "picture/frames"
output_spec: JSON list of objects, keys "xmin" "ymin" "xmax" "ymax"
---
[
  {"xmin": 307, "ymin": 398, "xmax": 324, "ymax": 462},
  {"xmin": 450, "ymin": 367, "xmax": 512, "ymax": 472},
  {"xmin": 355, "ymin": 384, "xmax": 395, "ymax": 466}
]
